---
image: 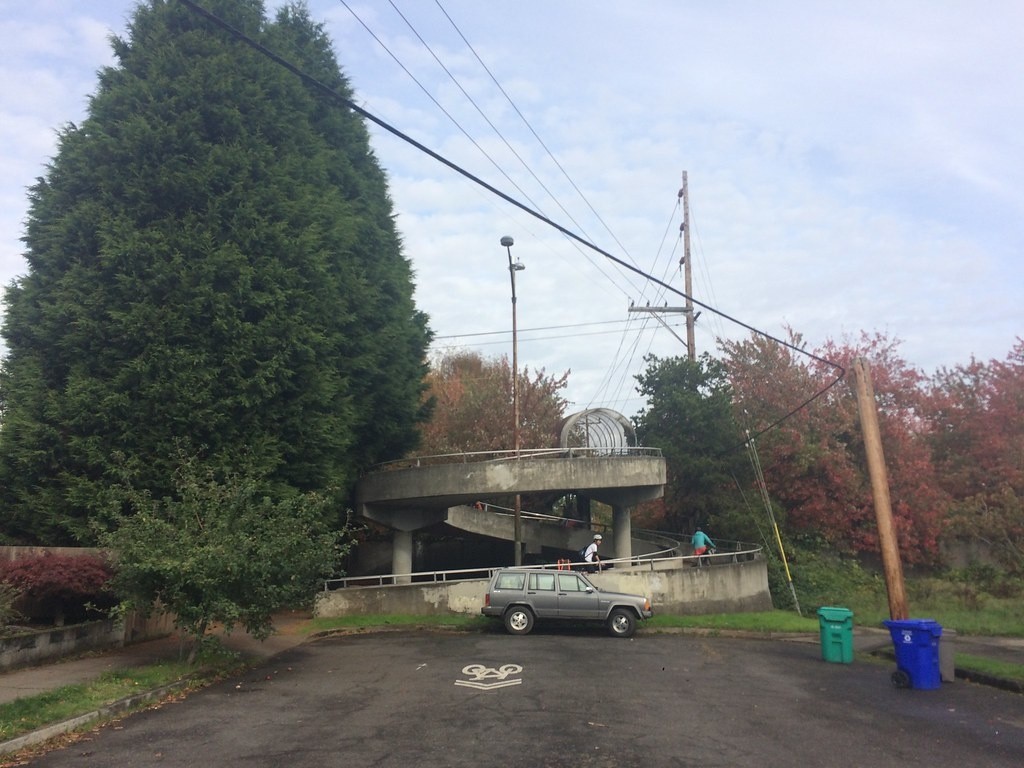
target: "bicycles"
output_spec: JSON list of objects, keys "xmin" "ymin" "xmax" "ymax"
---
[
  {"xmin": 689, "ymin": 541, "xmax": 716, "ymax": 567},
  {"xmin": 573, "ymin": 564, "xmax": 608, "ymax": 575}
]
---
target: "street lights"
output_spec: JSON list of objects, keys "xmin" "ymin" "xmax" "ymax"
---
[{"xmin": 499, "ymin": 235, "xmax": 527, "ymax": 567}]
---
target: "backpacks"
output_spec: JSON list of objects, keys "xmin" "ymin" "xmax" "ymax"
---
[{"xmin": 578, "ymin": 544, "xmax": 593, "ymax": 559}]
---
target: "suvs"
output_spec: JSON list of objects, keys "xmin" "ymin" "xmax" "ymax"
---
[{"xmin": 480, "ymin": 565, "xmax": 652, "ymax": 637}]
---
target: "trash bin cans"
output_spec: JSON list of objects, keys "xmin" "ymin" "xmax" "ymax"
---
[
  {"xmin": 882, "ymin": 618, "xmax": 943, "ymax": 690},
  {"xmin": 817, "ymin": 606, "xmax": 854, "ymax": 664}
]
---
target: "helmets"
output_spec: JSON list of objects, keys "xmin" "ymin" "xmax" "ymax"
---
[{"xmin": 593, "ymin": 534, "xmax": 603, "ymax": 540}]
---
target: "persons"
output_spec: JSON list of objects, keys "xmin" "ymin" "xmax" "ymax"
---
[
  {"xmin": 580, "ymin": 534, "xmax": 602, "ymax": 574},
  {"xmin": 691, "ymin": 526, "xmax": 716, "ymax": 566}
]
